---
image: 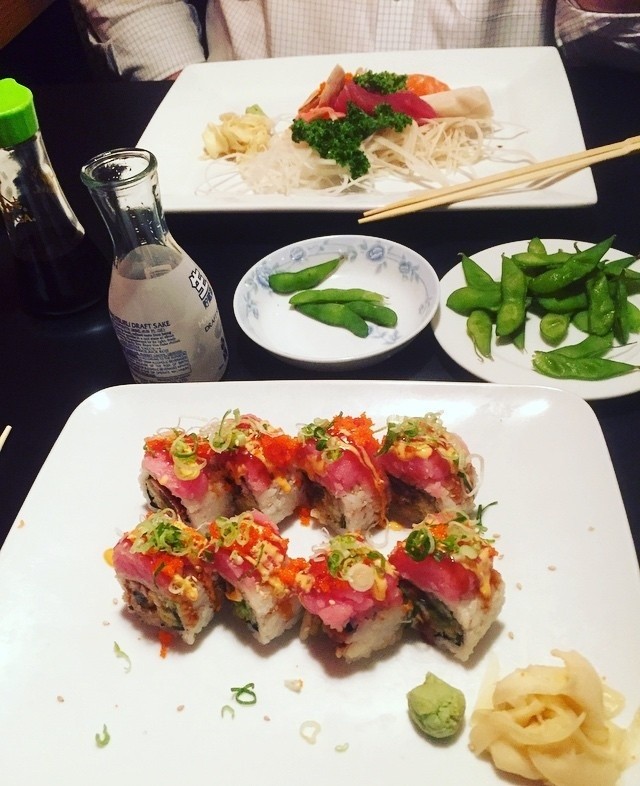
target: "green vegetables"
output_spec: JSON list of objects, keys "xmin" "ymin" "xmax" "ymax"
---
[{"xmin": 292, "ymin": 71, "xmax": 415, "ymax": 180}]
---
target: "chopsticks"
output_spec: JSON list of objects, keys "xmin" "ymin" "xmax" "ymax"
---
[{"xmin": 357, "ymin": 135, "xmax": 640, "ymax": 224}]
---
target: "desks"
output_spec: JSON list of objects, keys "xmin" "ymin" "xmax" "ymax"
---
[{"xmin": 1, "ymin": 79, "xmax": 639, "ymax": 552}]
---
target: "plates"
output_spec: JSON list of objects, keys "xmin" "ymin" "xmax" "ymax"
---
[
  {"xmin": 233, "ymin": 234, "xmax": 440, "ymax": 375},
  {"xmin": 117, "ymin": 47, "xmax": 598, "ymax": 212},
  {"xmin": 430, "ymin": 239, "xmax": 640, "ymax": 401},
  {"xmin": 1, "ymin": 379, "xmax": 639, "ymax": 784}
]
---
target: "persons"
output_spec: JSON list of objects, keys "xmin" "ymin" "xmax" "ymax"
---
[{"xmin": 80, "ymin": 0, "xmax": 640, "ymax": 81}]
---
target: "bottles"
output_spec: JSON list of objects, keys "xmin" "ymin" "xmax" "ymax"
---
[
  {"xmin": 79, "ymin": 146, "xmax": 228, "ymax": 384},
  {"xmin": 1, "ymin": 77, "xmax": 112, "ymax": 323}
]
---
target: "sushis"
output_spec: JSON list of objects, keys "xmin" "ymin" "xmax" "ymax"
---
[{"xmin": 109, "ymin": 412, "xmax": 507, "ymax": 661}]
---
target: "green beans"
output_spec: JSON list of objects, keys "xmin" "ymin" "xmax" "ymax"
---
[
  {"xmin": 447, "ymin": 233, "xmax": 640, "ymax": 381},
  {"xmin": 267, "ymin": 256, "xmax": 398, "ymax": 338}
]
